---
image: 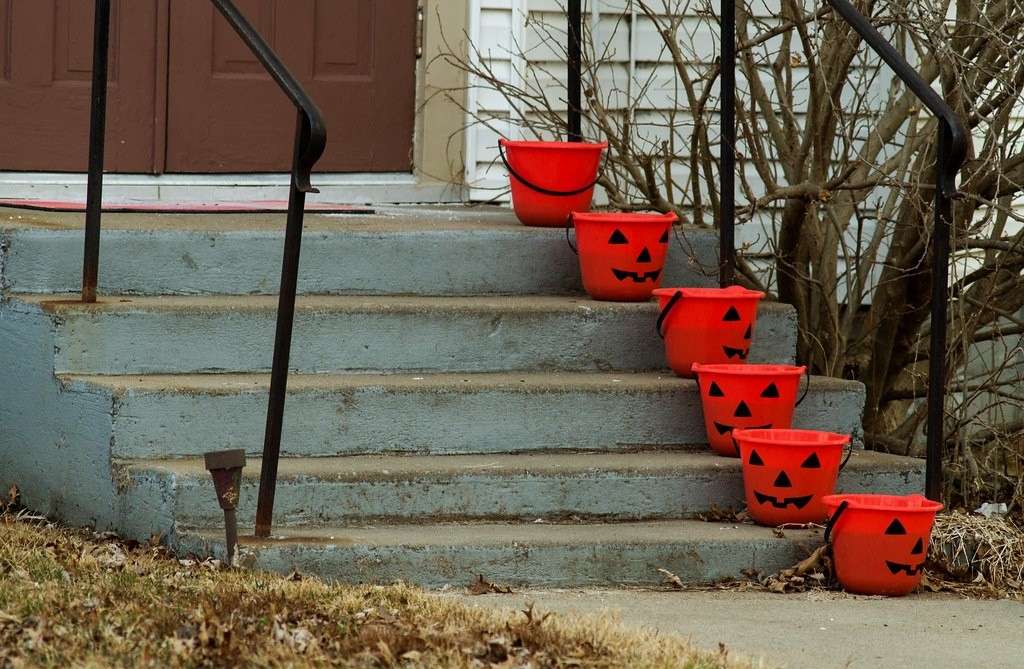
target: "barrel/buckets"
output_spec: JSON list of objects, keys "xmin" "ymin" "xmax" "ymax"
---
[
  {"xmin": 732, "ymin": 429, "xmax": 853, "ymax": 528},
  {"xmin": 497, "ymin": 139, "xmax": 608, "ymax": 225},
  {"xmin": 652, "ymin": 286, "xmax": 766, "ymax": 378},
  {"xmin": 566, "ymin": 211, "xmax": 678, "ymax": 301},
  {"xmin": 691, "ymin": 362, "xmax": 809, "ymax": 458},
  {"xmin": 820, "ymin": 494, "xmax": 944, "ymax": 597}
]
